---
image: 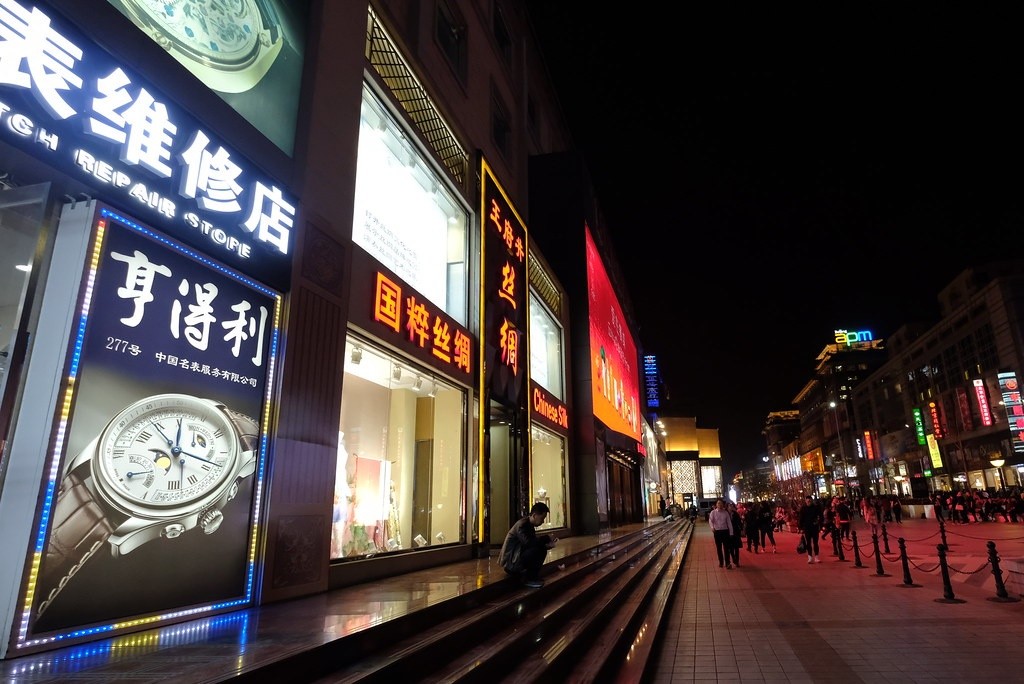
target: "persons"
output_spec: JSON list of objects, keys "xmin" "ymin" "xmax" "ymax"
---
[
  {"xmin": 709, "ymin": 498, "xmax": 733, "ymax": 569},
  {"xmin": 859, "ymin": 493, "xmax": 903, "ymax": 527},
  {"xmin": 724, "ymin": 502, "xmax": 746, "ymax": 568},
  {"xmin": 496, "ymin": 503, "xmax": 560, "ymax": 588},
  {"xmin": 660, "ymin": 498, "xmax": 666, "ymax": 516},
  {"xmin": 928, "ymin": 486, "xmax": 1024, "ymax": 527},
  {"xmin": 759, "ymin": 501, "xmax": 777, "ymax": 555},
  {"xmin": 665, "ymin": 496, "xmax": 671, "ymax": 510},
  {"xmin": 822, "ymin": 496, "xmax": 854, "ymax": 542},
  {"xmin": 797, "ymin": 495, "xmax": 824, "ymax": 564},
  {"xmin": 688, "ymin": 504, "xmax": 699, "ymax": 524},
  {"xmin": 771, "ymin": 506, "xmax": 789, "ymax": 532},
  {"xmin": 744, "ymin": 502, "xmax": 760, "ymax": 554}
]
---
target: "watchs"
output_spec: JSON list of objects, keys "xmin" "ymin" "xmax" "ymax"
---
[{"xmin": 28, "ymin": 394, "xmax": 260, "ymax": 627}]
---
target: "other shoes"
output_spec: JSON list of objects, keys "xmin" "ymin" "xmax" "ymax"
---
[
  {"xmin": 734, "ymin": 562, "xmax": 741, "ymax": 566},
  {"xmin": 719, "ymin": 563, "xmax": 723, "ymax": 567},
  {"xmin": 983, "ymin": 519, "xmax": 997, "ymax": 523},
  {"xmin": 808, "ymin": 554, "xmax": 813, "ymax": 563},
  {"xmin": 754, "ymin": 549, "xmax": 759, "ymax": 554},
  {"xmin": 814, "ymin": 555, "xmax": 821, "ymax": 563},
  {"xmin": 761, "ymin": 548, "xmax": 767, "ymax": 553},
  {"xmin": 974, "ymin": 521, "xmax": 980, "ymax": 524},
  {"xmin": 773, "ymin": 545, "xmax": 778, "ymax": 553},
  {"xmin": 953, "ymin": 522, "xmax": 970, "ymax": 525},
  {"xmin": 725, "ymin": 564, "xmax": 732, "ymax": 569},
  {"xmin": 524, "ymin": 578, "xmax": 545, "ymax": 588},
  {"xmin": 1004, "ymin": 519, "xmax": 1020, "ymax": 525},
  {"xmin": 747, "ymin": 547, "xmax": 751, "ymax": 552}
]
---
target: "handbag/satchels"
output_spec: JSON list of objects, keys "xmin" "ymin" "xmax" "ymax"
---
[{"xmin": 797, "ymin": 533, "xmax": 809, "ymax": 554}]
